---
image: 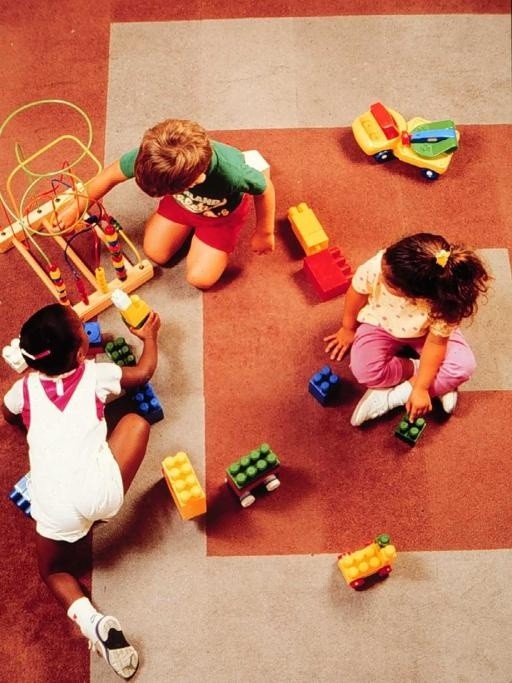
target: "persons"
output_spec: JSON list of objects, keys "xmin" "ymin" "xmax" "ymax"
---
[
  {"xmin": 320, "ymin": 230, "xmax": 495, "ymax": 429},
  {"xmin": 0, "ymin": 304, "xmax": 163, "ymax": 682},
  {"xmin": 49, "ymin": 116, "xmax": 278, "ymax": 292}
]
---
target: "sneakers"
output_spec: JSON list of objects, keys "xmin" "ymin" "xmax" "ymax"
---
[
  {"xmin": 349, "ymin": 383, "xmax": 395, "ymax": 427},
  {"xmin": 438, "ymin": 388, "xmax": 459, "ymax": 415},
  {"xmin": 88, "ymin": 614, "xmax": 140, "ymax": 680}
]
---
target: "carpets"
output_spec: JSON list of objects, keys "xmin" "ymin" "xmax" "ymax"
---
[{"xmin": 90, "ymin": 12, "xmax": 512, "ymax": 683}]
